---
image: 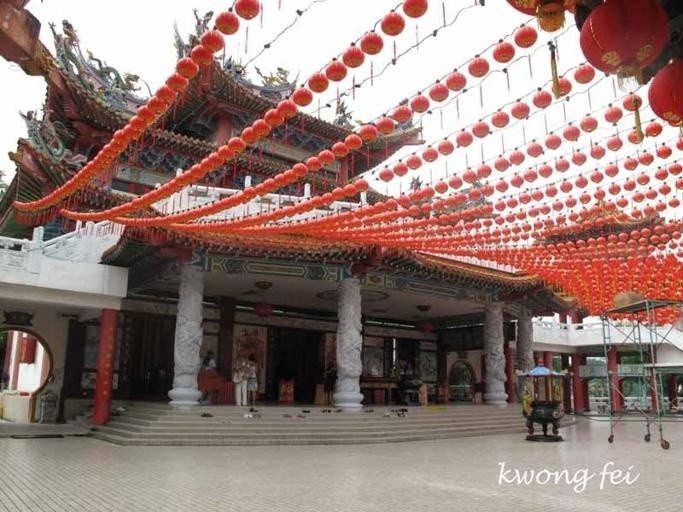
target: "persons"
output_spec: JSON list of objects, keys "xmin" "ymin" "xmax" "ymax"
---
[
  {"xmin": 322, "ymin": 358, "xmax": 337, "ymax": 406},
  {"xmin": 232, "ymin": 348, "xmax": 259, "ymax": 406},
  {"xmin": 198, "ymin": 350, "xmax": 218, "ymax": 404}
]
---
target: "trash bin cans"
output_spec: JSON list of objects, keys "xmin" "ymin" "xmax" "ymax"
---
[
  {"xmin": 39, "ymin": 393, "xmax": 58, "ymax": 423},
  {"xmin": 474, "ymin": 384, "xmax": 483, "ymax": 403}
]
